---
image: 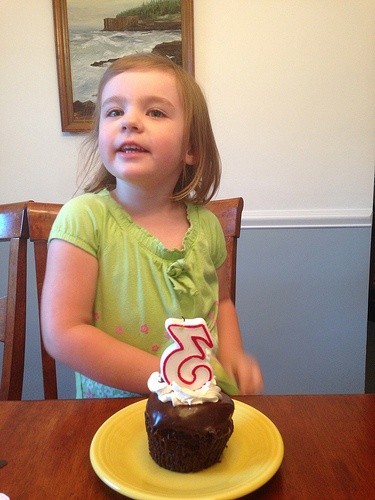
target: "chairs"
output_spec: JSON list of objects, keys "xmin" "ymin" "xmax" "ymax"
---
[{"xmin": 0, "ymin": 197, "xmax": 244, "ymax": 401}]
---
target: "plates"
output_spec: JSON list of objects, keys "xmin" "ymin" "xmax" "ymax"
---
[{"xmin": 90, "ymin": 398, "xmax": 284, "ymax": 500}]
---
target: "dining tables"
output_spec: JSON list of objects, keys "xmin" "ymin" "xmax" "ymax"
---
[{"xmin": 0, "ymin": 394, "xmax": 375, "ymax": 500}]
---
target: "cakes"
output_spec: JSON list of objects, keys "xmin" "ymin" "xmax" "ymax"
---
[{"xmin": 145, "ymin": 371, "xmax": 235, "ymax": 474}]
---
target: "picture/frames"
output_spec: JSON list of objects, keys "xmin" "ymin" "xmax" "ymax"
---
[{"xmin": 52, "ymin": 0, "xmax": 195, "ymax": 132}]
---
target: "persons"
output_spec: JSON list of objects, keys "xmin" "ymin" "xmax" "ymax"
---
[{"xmin": 41, "ymin": 53, "xmax": 263, "ymax": 399}]
